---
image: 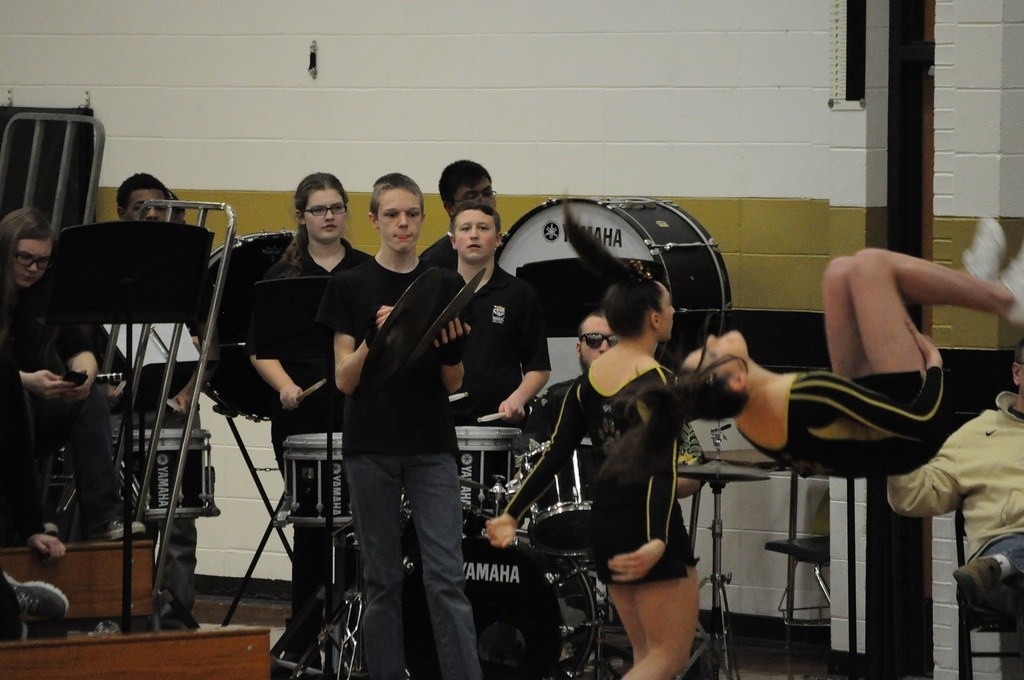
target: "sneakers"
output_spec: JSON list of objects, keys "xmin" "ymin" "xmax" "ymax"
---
[{"xmin": 1, "ymin": 572, "xmax": 71, "ymax": 622}]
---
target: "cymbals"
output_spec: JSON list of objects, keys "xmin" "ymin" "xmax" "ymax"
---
[
  {"xmin": 676, "ymin": 458, "xmax": 772, "ymax": 484},
  {"xmin": 358, "ymin": 264, "xmax": 487, "ymax": 397}
]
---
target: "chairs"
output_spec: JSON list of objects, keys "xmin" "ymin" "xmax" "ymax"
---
[
  {"xmin": 765, "ymin": 537, "xmax": 830, "ymax": 626},
  {"xmin": 952, "ymin": 498, "xmax": 1024, "ymax": 680}
]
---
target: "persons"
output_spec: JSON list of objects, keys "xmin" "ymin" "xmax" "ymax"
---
[
  {"xmin": 885, "ymin": 339, "xmax": 1024, "ymax": 611},
  {"xmin": 315, "ymin": 173, "xmax": 483, "ymax": 680},
  {"xmin": 526, "ymin": 310, "xmax": 705, "ymax": 625},
  {"xmin": 680, "ymin": 218, "xmax": 1024, "ymax": 478},
  {"xmin": 486, "ymin": 224, "xmax": 699, "ymax": 680},
  {"xmin": 246, "ymin": 171, "xmax": 374, "ymax": 669},
  {"xmin": 449, "ymin": 199, "xmax": 552, "ymax": 481},
  {"xmin": 0, "ymin": 174, "xmax": 217, "ymax": 642},
  {"xmin": 419, "ymin": 159, "xmax": 496, "ymax": 270}
]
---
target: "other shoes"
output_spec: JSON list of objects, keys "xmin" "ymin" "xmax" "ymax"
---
[
  {"xmin": 952, "ymin": 558, "xmax": 1003, "ymax": 609},
  {"xmin": 959, "ymin": 216, "xmax": 1008, "ymax": 282},
  {"xmin": 998, "ymin": 240, "xmax": 1024, "ymax": 328},
  {"xmin": 42, "ymin": 521, "xmax": 60, "ymax": 537},
  {"xmin": 91, "ymin": 519, "xmax": 147, "ymax": 541}
]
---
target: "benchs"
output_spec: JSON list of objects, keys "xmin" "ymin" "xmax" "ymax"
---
[{"xmin": 0, "ymin": 539, "xmax": 270, "ymax": 680}]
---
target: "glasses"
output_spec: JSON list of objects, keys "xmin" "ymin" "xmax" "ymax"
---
[
  {"xmin": 304, "ymin": 204, "xmax": 348, "ymax": 216},
  {"xmin": 453, "ymin": 191, "xmax": 497, "ymax": 203},
  {"xmin": 15, "ymin": 250, "xmax": 55, "ymax": 269},
  {"xmin": 578, "ymin": 332, "xmax": 618, "ymax": 348}
]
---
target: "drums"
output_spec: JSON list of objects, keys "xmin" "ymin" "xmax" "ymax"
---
[
  {"xmin": 403, "ymin": 529, "xmax": 600, "ymax": 680},
  {"xmin": 403, "ymin": 425, "xmax": 524, "ymax": 540},
  {"xmin": 189, "ymin": 229, "xmax": 302, "ymax": 421},
  {"xmin": 109, "ymin": 427, "xmax": 212, "ymax": 522},
  {"xmin": 519, "ymin": 435, "xmax": 594, "ymax": 559},
  {"xmin": 279, "ymin": 432, "xmax": 354, "ymax": 528},
  {"xmin": 492, "ymin": 196, "xmax": 737, "ymax": 405}
]
